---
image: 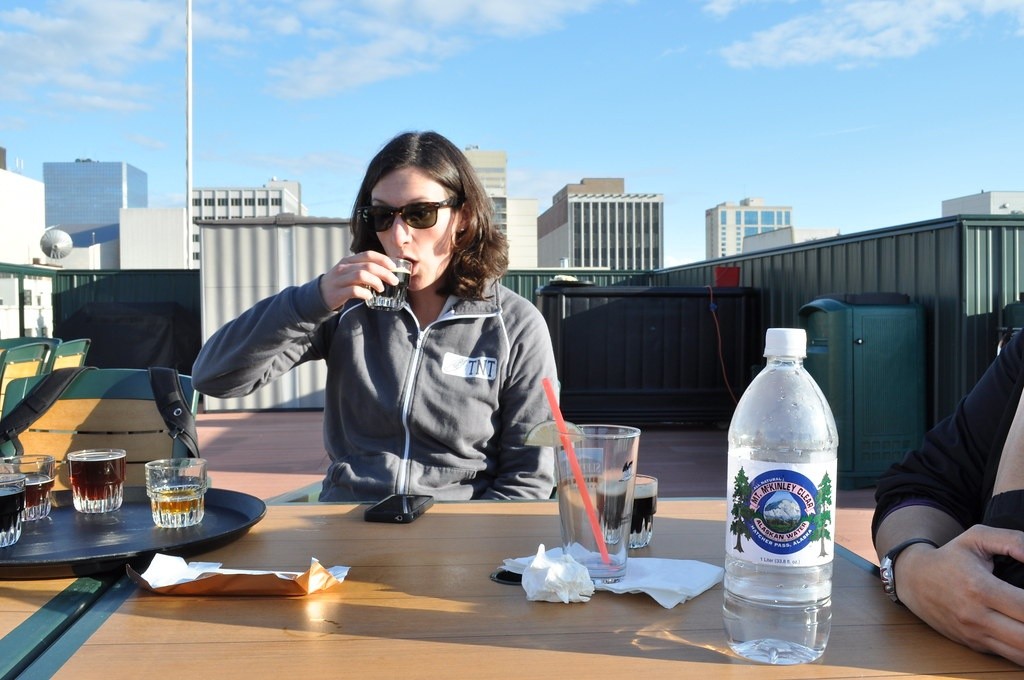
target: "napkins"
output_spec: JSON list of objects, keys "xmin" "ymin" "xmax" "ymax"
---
[{"xmin": 498, "ymin": 542, "xmax": 725, "ymax": 610}]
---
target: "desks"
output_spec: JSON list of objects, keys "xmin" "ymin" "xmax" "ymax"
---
[
  {"xmin": 15, "ymin": 496, "xmax": 1024, "ymax": 680},
  {"xmin": 0, "ymin": 578, "xmax": 109, "ymax": 680}
]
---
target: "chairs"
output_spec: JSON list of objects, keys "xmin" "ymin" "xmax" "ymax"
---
[
  {"xmin": 0, "ymin": 368, "xmax": 200, "ymax": 492},
  {"xmin": 0, "ymin": 331, "xmax": 91, "ymax": 420}
]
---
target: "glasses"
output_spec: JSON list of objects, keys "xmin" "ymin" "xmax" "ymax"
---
[{"xmin": 356, "ymin": 196, "xmax": 458, "ymax": 232}]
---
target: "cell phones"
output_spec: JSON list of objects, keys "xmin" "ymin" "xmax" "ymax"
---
[{"xmin": 364, "ymin": 494, "xmax": 434, "ymax": 524}]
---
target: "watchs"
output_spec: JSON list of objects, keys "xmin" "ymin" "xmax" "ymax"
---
[{"xmin": 880, "ymin": 538, "xmax": 939, "ymax": 606}]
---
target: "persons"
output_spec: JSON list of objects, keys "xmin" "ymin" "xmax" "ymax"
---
[
  {"xmin": 191, "ymin": 131, "xmax": 560, "ymax": 502},
  {"xmin": 872, "ymin": 330, "xmax": 1024, "ymax": 667}
]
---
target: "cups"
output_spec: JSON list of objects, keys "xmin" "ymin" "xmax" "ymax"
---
[
  {"xmin": 554, "ymin": 424, "xmax": 641, "ymax": 578},
  {"xmin": 0, "ymin": 455, "xmax": 55, "ymax": 522},
  {"xmin": 595, "ymin": 474, "xmax": 658, "ymax": 548},
  {"xmin": 66, "ymin": 448, "xmax": 126, "ymax": 514},
  {"xmin": 366, "ymin": 256, "xmax": 413, "ymax": 311},
  {"xmin": 145, "ymin": 457, "xmax": 208, "ymax": 528},
  {"xmin": 0, "ymin": 473, "xmax": 26, "ymax": 547}
]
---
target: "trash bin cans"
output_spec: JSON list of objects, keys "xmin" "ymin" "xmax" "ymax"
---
[{"xmin": 797, "ymin": 292, "xmax": 927, "ymax": 492}]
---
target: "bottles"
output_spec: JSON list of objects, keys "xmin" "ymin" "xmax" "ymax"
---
[{"xmin": 721, "ymin": 328, "xmax": 839, "ymax": 666}]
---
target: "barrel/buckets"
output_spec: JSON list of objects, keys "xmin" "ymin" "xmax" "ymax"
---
[{"xmin": 713, "ymin": 266, "xmax": 740, "ymax": 287}]
